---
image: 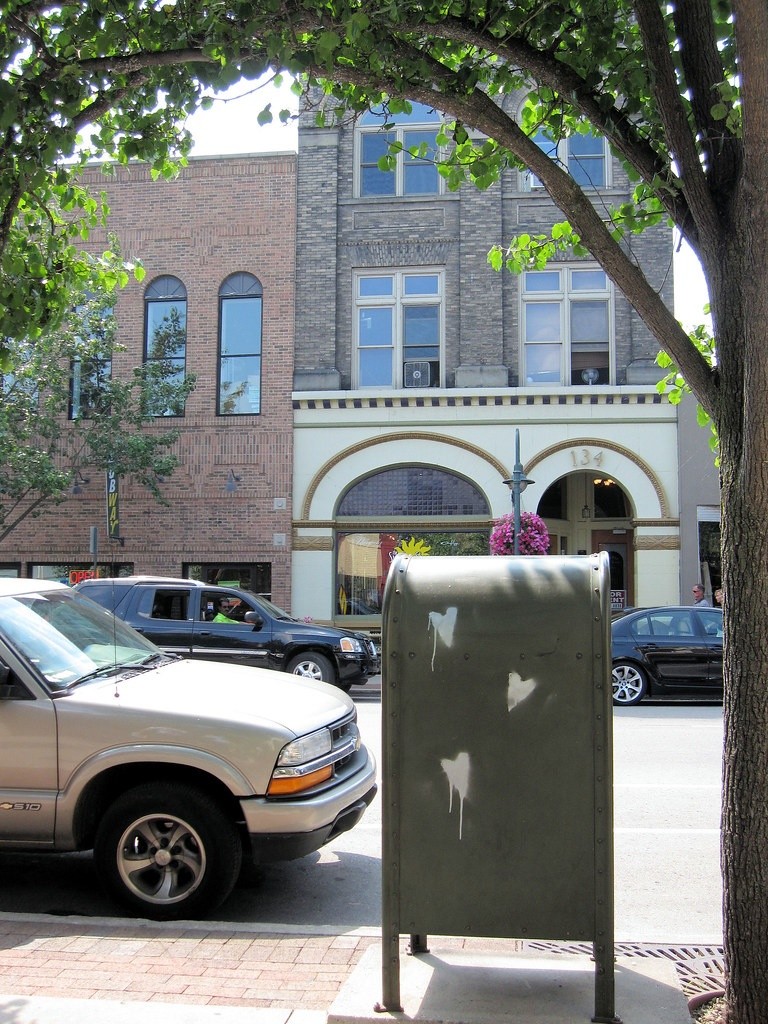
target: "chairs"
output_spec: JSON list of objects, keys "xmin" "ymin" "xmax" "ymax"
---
[
  {"xmin": 200, "ymin": 597, "xmax": 208, "ymax": 622},
  {"xmin": 153, "ymin": 603, "xmax": 169, "ymax": 619}
]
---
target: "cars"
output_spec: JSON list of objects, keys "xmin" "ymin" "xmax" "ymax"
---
[
  {"xmin": 611, "ymin": 604, "xmax": 724, "ymax": 706},
  {"xmin": 338, "ymin": 598, "xmax": 378, "ymax": 616},
  {"xmin": 0, "ymin": 577, "xmax": 377, "ymax": 922}
]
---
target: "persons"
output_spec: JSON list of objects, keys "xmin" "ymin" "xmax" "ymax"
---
[
  {"xmin": 212, "ymin": 598, "xmax": 256, "ymax": 625},
  {"xmin": 692, "ymin": 583, "xmax": 710, "ymax": 607},
  {"xmin": 714, "ymin": 589, "xmax": 724, "ymax": 609}
]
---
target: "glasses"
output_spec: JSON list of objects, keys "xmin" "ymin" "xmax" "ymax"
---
[
  {"xmin": 693, "ymin": 589, "xmax": 701, "ymax": 593},
  {"xmin": 221, "ymin": 606, "xmax": 231, "ymax": 609}
]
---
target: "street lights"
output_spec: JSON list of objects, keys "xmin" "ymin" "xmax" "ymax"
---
[{"xmin": 502, "ymin": 428, "xmax": 535, "ymax": 556}]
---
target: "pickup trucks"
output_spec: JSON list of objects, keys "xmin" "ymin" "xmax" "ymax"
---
[{"xmin": 71, "ymin": 572, "xmax": 378, "ymax": 696}]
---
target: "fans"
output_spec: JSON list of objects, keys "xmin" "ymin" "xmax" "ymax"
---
[{"xmin": 581, "ymin": 368, "xmax": 599, "ymax": 385}]
